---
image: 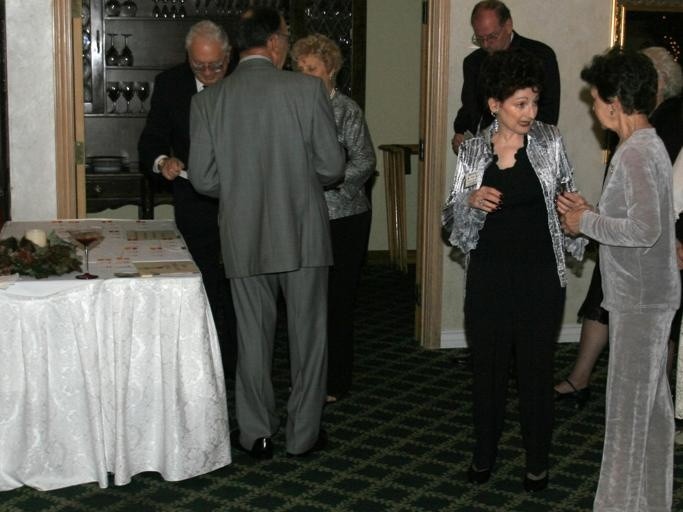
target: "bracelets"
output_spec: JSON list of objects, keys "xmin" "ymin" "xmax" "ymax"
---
[{"xmin": 158, "ymin": 159, "xmax": 163, "ymax": 167}]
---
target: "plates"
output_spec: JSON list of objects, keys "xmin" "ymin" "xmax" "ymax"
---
[
  {"xmin": 86, "ymin": 154, "xmax": 139, "ymax": 173},
  {"xmin": 80, "ymin": 3, "xmax": 93, "ymax": 103}
]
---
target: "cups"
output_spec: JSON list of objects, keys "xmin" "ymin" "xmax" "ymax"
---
[{"xmin": 23, "ymin": 229, "xmax": 47, "ymax": 247}]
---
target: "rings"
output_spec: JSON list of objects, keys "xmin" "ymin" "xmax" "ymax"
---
[{"xmin": 479, "ymin": 200, "xmax": 485, "ymax": 206}]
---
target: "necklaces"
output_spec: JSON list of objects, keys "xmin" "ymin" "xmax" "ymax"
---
[{"xmin": 330, "ymin": 88, "xmax": 335, "ymax": 101}]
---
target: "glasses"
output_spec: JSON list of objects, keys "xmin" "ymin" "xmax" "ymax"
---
[
  {"xmin": 469, "ymin": 25, "xmax": 507, "ymax": 46},
  {"xmin": 186, "ymin": 52, "xmax": 230, "ymax": 73}
]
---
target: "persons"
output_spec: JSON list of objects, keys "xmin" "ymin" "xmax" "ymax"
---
[
  {"xmin": 139, "ymin": 20, "xmax": 249, "ymax": 377},
  {"xmin": 441, "ymin": 66, "xmax": 588, "ymax": 495},
  {"xmin": 186, "ymin": 5, "xmax": 345, "ymax": 461},
  {"xmin": 580, "ymin": 46, "xmax": 683, "ymax": 512},
  {"xmin": 290, "ymin": 33, "xmax": 380, "ymax": 398},
  {"xmin": 452, "ymin": 0, "xmax": 560, "ymax": 157},
  {"xmin": 553, "ymin": 44, "xmax": 683, "ymax": 412}
]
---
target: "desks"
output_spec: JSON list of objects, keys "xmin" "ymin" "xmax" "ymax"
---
[{"xmin": 83, "ymin": 161, "xmax": 194, "ymax": 220}]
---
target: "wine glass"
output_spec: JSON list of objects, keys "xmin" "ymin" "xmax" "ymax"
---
[
  {"xmin": 104, "ymin": 33, "xmax": 133, "ymax": 67},
  {"xmin": 150, "ymin": 1, "xmax": 184, "ymax": 19},
  {"xmin": 67, "ymin": 226, "xmax": 106, "ymax": 279},
  {"xmin": 105, "ymin": 82, "xmax": 150, "ymax": 115},
  {"xmin": 191, "ymin": 1, "xmax": 284, "ymax": 17}
]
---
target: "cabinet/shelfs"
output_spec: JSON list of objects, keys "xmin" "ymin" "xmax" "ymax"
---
[{"xmin": 77, "ymin": 1, "xmax": 367, "ymax": 119}]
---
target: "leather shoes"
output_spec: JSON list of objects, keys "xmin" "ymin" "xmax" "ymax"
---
[
  {"xmin": 553, "ymin": 377, "xmax": 592, "ymax": 411},
  {"xmin": 227, "ymin": 428, "xmax": 274, "ymax": 461},
  {"xmin": 324, "ymin": 384, "xmax": 350, "ymax": 406},
  {"xmin": 467, "ymin": 461, "xmax": 492, "ymax": 485},
  {"xmin": 283, "ymin": 447, "xmax": 314, "ymax": 459},
  {"xmin": 523, "ymin": 469, "xmax": 551, "ymax": 492}
]
